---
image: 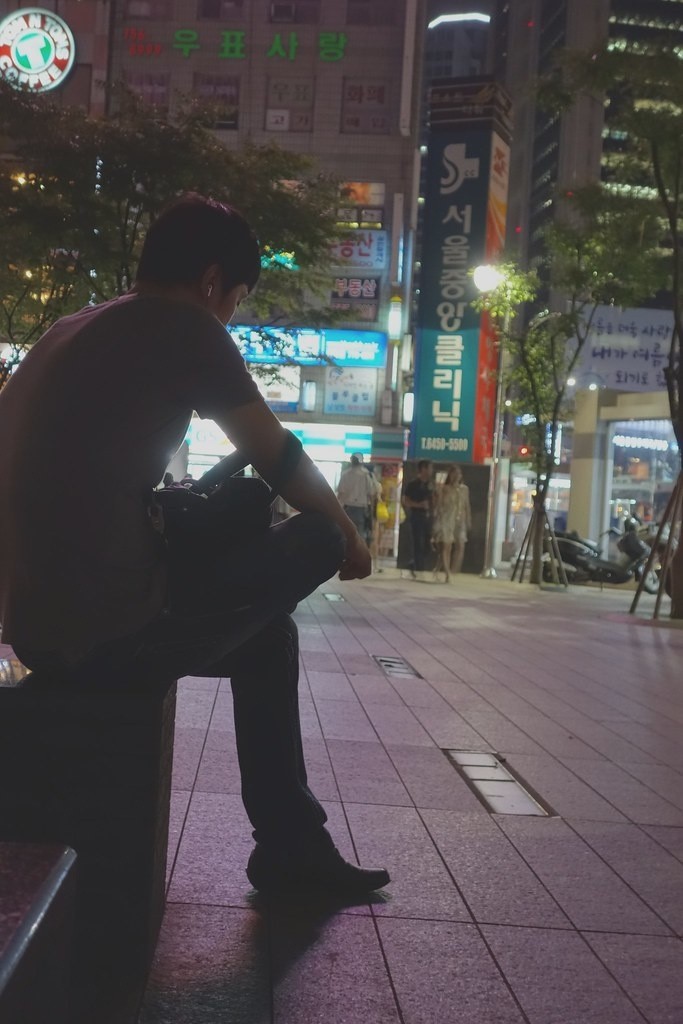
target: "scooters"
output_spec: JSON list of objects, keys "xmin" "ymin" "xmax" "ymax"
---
[{"xmin": 541, "ymin": 510, "xmax": 660, "ymax": 597}]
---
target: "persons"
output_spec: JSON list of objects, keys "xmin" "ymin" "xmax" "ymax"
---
[
  {"xmin": 1, "ymin": 191, "xmax": 391, "ymax": 899},
  {"xmin": 163, "ymin": 456, "xmax": 472, "ymax": 583}
]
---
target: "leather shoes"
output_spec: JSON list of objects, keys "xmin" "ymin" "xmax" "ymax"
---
[{"xmin": 246, "ymin": 828, "xmax": 390, "ymax": 899}]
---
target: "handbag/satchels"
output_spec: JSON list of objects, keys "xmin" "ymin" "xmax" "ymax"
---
[
  {"xmin": 375, "ymin": 501, "xmax": 390, "ymax": 524},
  {"xmin": 154, "ymin": 430, "xmax": 304, "ymax": 577}
]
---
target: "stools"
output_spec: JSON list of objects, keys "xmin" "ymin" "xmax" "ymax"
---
[
  {"xmin": 0, "ymin": 657, "xmax": 178, "ymax": 976},
  {"xmin": 0, "ymin": 840, "xmax": 82, "ymax": 1024}
]
